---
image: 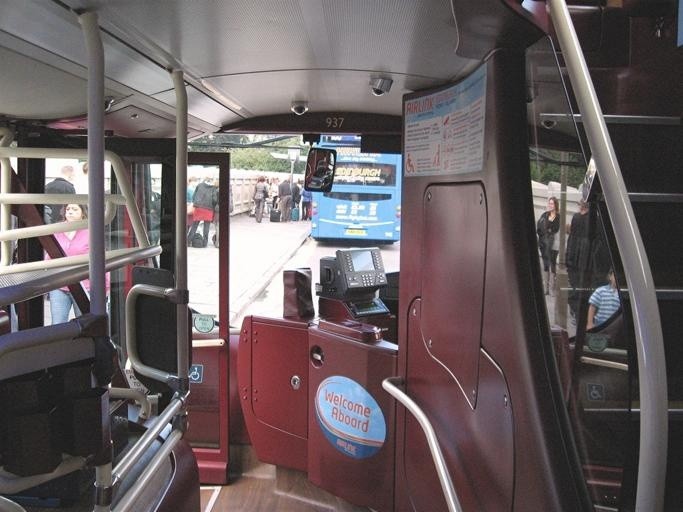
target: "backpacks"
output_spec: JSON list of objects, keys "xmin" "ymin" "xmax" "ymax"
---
[{"xmin": 191, "ymin": 232, "xmax": 204, "ymax": 248}]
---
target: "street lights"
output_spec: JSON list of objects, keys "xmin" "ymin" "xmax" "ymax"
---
[{"xmin": 288, "ymin": 146, "xmax": 300, "ymax": 192}]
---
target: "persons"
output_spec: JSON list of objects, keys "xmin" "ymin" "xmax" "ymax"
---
[
  {"xmin": 44, "ymin": 162, "xmax": 110, "ymax": 325},
  {"xmin": 187, "ymin": 175, "xmax": 234, "ymax": 248},
  {"xmin": 252, "ymin": 176, "xmax": 311, "ymax": 223},
  {"xmin": 537, "ymin": 196, "xmax": 620, "ymax": 330}
]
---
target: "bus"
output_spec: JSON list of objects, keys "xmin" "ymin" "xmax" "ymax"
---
[{"xmin": 311, "ymin": 134, "xmax": 402, "ymax": 241}]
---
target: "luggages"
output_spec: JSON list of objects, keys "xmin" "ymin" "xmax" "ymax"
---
[
  {"xmin": 291, "ymin": 207, "xmax": 299, "ymax": 222},
  {"xmin": 270, "ymin": 208, "xmax": 281, "ymax": 223}
]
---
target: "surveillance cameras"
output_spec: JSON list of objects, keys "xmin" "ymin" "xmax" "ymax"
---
[
  {"xmin": 290, "ymin": 102, "xmax": 309, "ymax": 115},
  {"xmin": 369, "ymin": 78, "xmax": 393, "ymax": 96}
]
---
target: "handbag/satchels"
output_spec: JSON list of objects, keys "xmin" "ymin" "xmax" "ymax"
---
[{"xmin": 263, "ymin": 187, "xmax": 270, "ymax": 199}]
